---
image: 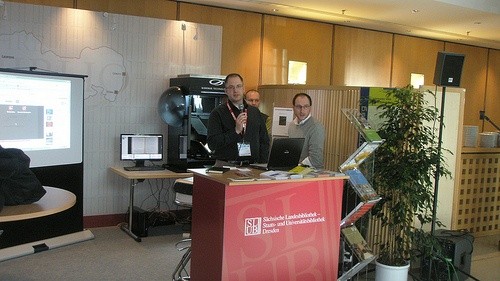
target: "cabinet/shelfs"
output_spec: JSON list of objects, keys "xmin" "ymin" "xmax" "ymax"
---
[{"xmin": 167, "ymin": 78, "xmax": 228, "ymax": 169}]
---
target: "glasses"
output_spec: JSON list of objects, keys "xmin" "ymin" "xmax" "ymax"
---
[
  {"xmin": 225, "ymin": 84, "xmax": 243, "ymax": 90},
  {"xmin": 294, "ymin": 105, "xmax": 312, "ymax": 109}
]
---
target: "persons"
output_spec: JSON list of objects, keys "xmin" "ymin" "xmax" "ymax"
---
[
  {"xmin": 288, "ymin": 92, "xmax": 325, "ymax": 172},
  {"xmin": 206, "ymin": 73, "xmax": 274, "ymax": 170}
]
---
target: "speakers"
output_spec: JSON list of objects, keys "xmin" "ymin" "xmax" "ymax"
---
[
  {"xmin": 433, "ymin": 52, "xmax": 465, "ymax": 88},
  {"xmin": 421, "ymin": 229, "xmax": 474, "ymax": 281}
]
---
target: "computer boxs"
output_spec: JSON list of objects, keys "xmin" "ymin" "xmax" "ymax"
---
[{"xmin": 128, "ymin": 206, "xmax": 147, "ymax": 237}]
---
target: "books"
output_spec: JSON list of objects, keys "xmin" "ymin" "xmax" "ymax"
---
[{"xmin": 208, "ymin": 165, "xmax": 345, "ymax": 181}]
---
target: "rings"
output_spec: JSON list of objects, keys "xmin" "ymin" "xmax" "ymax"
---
[{"xmin": 240, "ymin": 119, "xmax": 243, "ymax": 122}]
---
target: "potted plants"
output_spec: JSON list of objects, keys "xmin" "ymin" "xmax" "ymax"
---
[{"xmin": 361, "ymin": 86, "xmax": 454, "ymax": 281}]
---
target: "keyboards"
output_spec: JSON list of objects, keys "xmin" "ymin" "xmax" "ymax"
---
[{"xmin": 124, "ymin": 166, "xmax": 165, "ymax": 170}]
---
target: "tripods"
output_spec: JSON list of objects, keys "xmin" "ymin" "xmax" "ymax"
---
[{"xmin": 404, "ymin": 87, "xmax": 480, "ymax": 281}]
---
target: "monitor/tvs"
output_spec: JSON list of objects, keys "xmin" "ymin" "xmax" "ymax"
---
[{"xmin": 120, "ymin": 134, "xmax": 163, "ymax": 167}]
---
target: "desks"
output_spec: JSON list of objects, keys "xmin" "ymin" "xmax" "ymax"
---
[
  {"xmin": 0, "ymin": 186, "xmax": 77, "ymax": 222},
  {"xmin": 110, "ymin": 165, "xmax": 193, "ymax": 243}
]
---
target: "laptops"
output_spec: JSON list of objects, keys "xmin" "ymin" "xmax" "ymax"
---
[{"xmin": 250, "ymin": 138, "xmax": 305, "ymax": 170}]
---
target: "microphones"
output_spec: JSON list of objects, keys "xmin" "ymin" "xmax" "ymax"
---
[{"xmin": 239, "ymin": 104, "xmax": 245, "ymax": 128}]
